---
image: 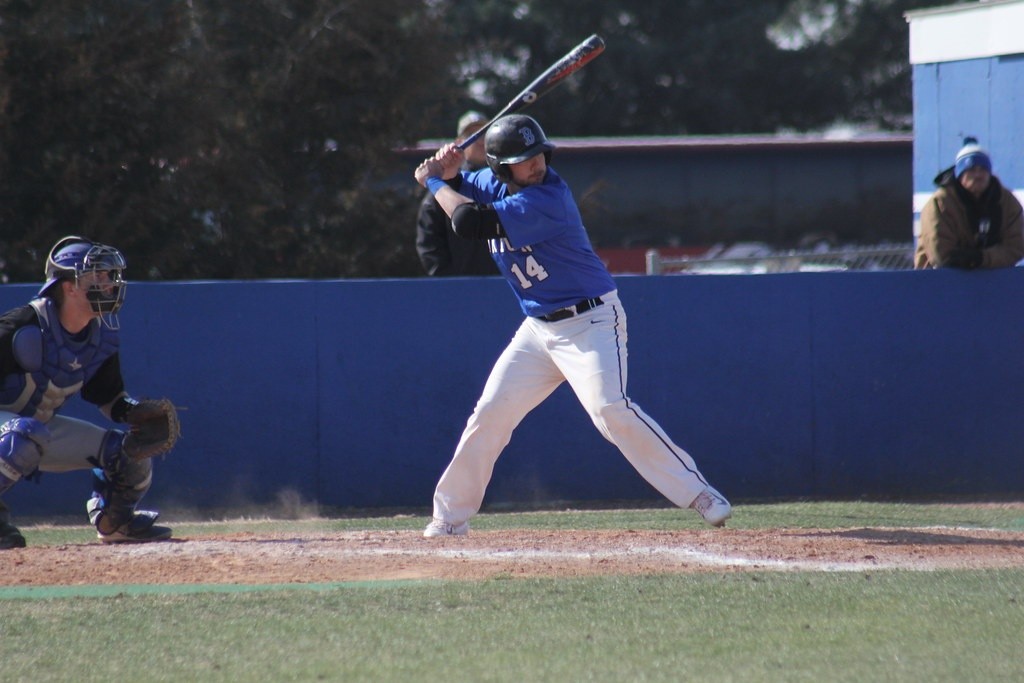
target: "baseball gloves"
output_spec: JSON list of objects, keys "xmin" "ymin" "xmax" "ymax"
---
[{"xmin": 120, "ymin": 395, "xmax": 185, "ymax": 466}]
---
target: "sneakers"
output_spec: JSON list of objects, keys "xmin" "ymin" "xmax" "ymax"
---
[
  {"xmin": 0, "ymin": 521, "xmax": 26, "ymax": 550},
  {"xmin": 690, "ymin": 486, "xmax": 731, "ymax": 526},
  {"xmin": 97, "ymin": 513, "xmax": 173, "ymax": 545},
  {"xmin": 421, "ymin": 519, "xmax": 467, "ymax": 539}
]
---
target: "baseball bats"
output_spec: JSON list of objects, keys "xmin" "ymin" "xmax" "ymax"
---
[{"xmin": 457, "ymin": 32, "xmax": 607, "ymax": 151}]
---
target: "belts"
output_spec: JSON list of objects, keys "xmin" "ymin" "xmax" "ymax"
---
[{"xmin": 535, "ymin": 296, "xmax": 604, "ymax": 322}]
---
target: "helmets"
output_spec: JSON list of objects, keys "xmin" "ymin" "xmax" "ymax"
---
[
  {"xmin": 483, "ymin": 114, "xmax": 554, "ymax": 184},
  {"xmin": 37, "ymin": 242, "xmax": 116, "ymax": 298}
]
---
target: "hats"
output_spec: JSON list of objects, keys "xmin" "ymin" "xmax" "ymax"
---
[
  {"xmin": 955, "ymin": 136, "xmax": 991, "ymax": 181},
  {"xmin": 456, "ymin": 112, "xmax": 490, "ymax": 135}
]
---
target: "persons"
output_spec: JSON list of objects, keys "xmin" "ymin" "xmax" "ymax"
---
[
  {"xmin": 413, "ymin": 113, "xmax": 736, "ymax": 539},
  {"xmin": 0, "ymin": 234, "xmax": 189, "ymax": 549},
  {"xmin": 418, "ymin": 112, "xmax": 503, "ymax": 279},
  {"xmin": 915, "ymin": 132, "xmax": 1024, "ymax": 269}
]
